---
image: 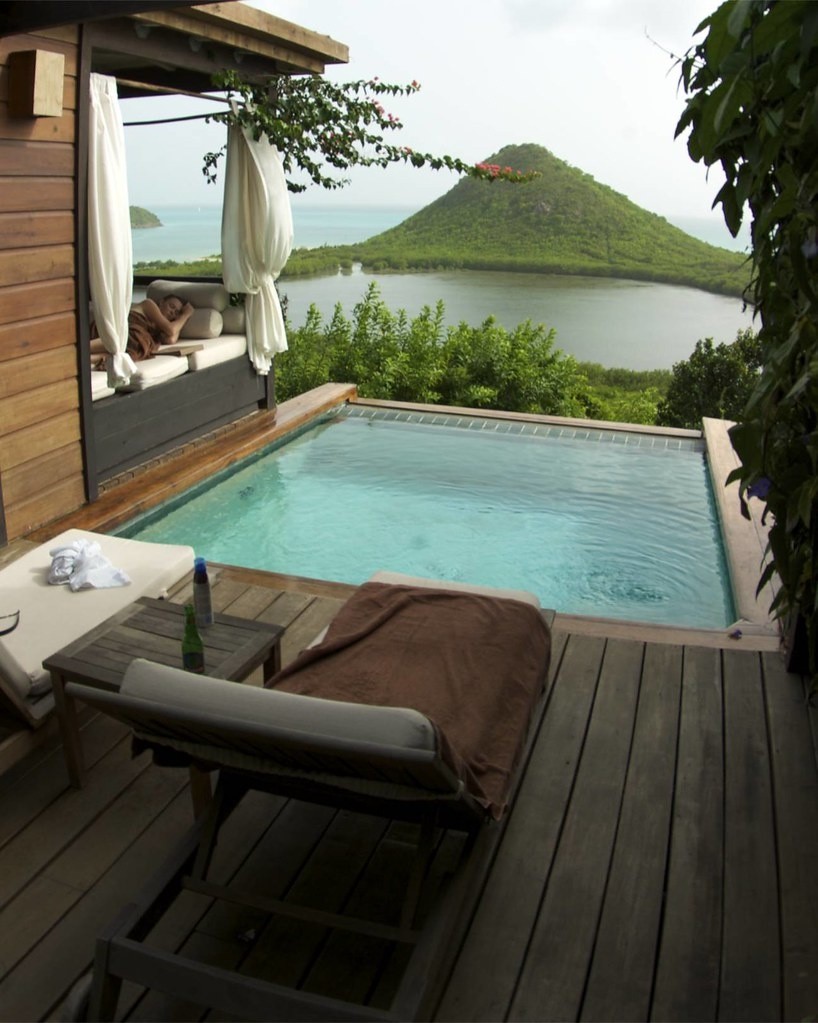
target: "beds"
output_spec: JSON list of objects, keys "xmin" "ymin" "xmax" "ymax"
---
[{"xmin": 91, "ymin": 278, "xmax": 251, "ymax": 399}]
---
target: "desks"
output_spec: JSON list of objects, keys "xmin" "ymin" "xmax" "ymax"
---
[{"xmin": 46, "ymin": 596, "xmax": 285, "ymax": 824}]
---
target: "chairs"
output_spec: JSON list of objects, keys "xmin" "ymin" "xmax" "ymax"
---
[
  {"xmin": 65, "ymin": 579, "xmax": 554, "ymax": 1020},
  {"xmin": 0, "ymin": 525, "xmax": 200, "ymax": 744}
]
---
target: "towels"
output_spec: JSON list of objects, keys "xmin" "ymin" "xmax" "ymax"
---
[{"xmin": 46, "ymin": 538, "xmax": 133, "ymax": 592}]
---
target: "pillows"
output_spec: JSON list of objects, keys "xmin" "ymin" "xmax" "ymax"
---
[{"xmin": 146, "ymin": 279, "xmax": 249, "ymax": 339}]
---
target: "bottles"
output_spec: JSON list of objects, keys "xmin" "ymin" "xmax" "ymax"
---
[
  {"xmin": 181, "ymin": 603, "xmax": 205, "ymax": 674},
  {"xmin": 193, "ymin": 557, "xmax": 215, "ymax": 628}
]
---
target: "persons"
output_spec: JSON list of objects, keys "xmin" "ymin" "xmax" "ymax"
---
[{"xmin": 89, "ymin": 294, "xmax": 194, "ymax": 371}]
